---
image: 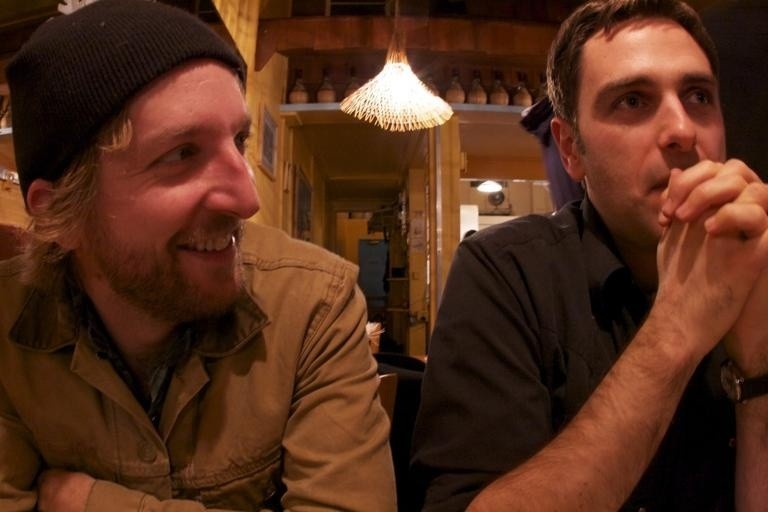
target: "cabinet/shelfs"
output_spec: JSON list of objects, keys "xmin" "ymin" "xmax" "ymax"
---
[{"xmin": 387, "ymin": 277, "xmax": 409, "ymax": 312}]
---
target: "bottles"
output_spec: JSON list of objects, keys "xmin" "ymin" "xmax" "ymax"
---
[{"xmin": 288, "ymin": 66, "xmax": 533, "ymax": 106}]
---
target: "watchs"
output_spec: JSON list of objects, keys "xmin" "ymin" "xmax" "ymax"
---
[{"xmin": 720, "ymin": 358, "xmax": 768, "ymax": 404}]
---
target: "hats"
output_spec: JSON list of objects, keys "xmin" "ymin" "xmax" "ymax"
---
[{"xmin": 0, "ymin": 0, "xmax": 247, "ymax": 207}]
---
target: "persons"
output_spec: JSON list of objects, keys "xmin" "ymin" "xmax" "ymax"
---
[
  {"xmin": 0, "ymin": 0, "xmax": 398, "ymax": 512},
  {"xmin": 408, "ymin": 0, "xmax": 768, "ymax": 512}
]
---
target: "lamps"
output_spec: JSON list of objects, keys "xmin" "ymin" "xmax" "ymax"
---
[{"xmin": 338, "ymin": 0, "xmax": 454, "ymax": 132}]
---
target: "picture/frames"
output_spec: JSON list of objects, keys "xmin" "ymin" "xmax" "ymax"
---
[
  {"xmin": 258, "ymin": 103, "xmax": 279, "ymax": 182},
  {"xmin": 293, "ymin": 163, "xmax": 314, "ymax": 242}
]
